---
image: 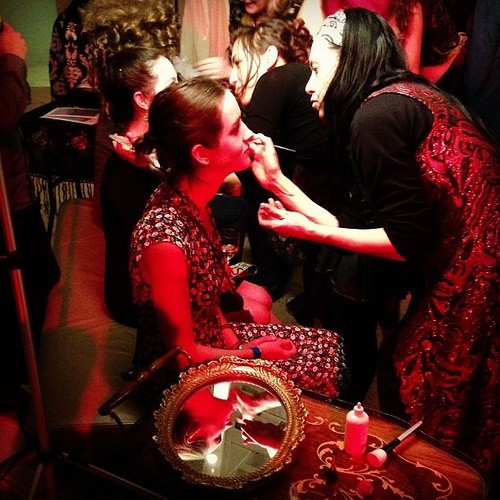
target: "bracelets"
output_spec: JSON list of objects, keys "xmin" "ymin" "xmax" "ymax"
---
[{"xmin": 251, "ymin": 347, "xmax": 261, "ymax": 358}]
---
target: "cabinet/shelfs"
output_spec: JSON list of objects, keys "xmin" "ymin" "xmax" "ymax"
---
[{"xmin": 18, "ymin": 96, "xmax": 97, "ymax": 239}]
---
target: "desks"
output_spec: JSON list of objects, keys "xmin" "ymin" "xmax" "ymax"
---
[{"xmin": 114, "ymin": 388, "xmax": 491, "ymax": 500}]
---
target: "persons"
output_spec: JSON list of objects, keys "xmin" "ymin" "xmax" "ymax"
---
[
  {"xmin": 247, "ymin": 6, "xmax": 500, "ymax": 480},
  {"xmin": 0, "ymin": 22, "xmax": 62, "ymax": 459},
  {"xmin": 128, "ymin": 78, "xmax": 352, "ymax": 400},
  {"xmin": 47, "ymin": 0, "xmax": 499, "ymax": 329}
]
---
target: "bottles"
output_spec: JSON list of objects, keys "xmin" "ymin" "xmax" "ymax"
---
[{"xmin": 343, "ymin": 401, "xmax": 370, "ymax": 458}]
